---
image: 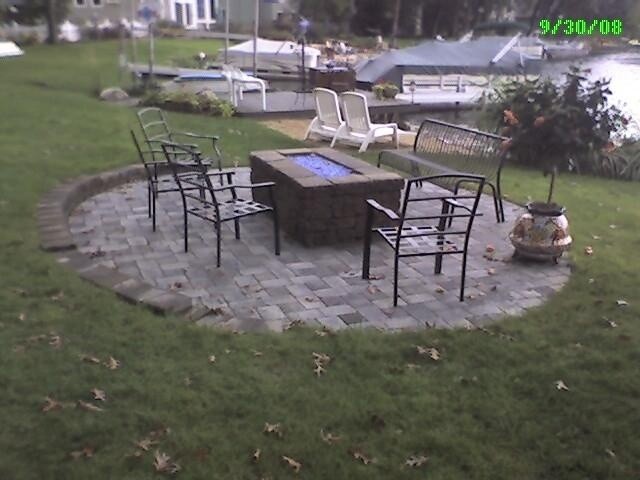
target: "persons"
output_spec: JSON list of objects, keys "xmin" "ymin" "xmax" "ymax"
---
[
  {"xmin": 297, "ymin": 15, "xmax": 311, "ymax": 45},
  {"xmin": 374, "ymin": 32, "xmax": 383, "ymax": 51}
]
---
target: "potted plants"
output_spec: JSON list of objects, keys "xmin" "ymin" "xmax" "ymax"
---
[{"xmin": 481, "ymin": 62, "xmax": 640, "ymax": 265}]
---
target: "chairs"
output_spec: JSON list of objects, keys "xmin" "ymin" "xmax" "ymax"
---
[
  {"xmin": 303, "ymin": 87, "xmax": 418, "ymax": 154},
  {"xmin": 130, "ymin": 106, "xmax": 281, "ymax": 268},
  {"xmin": 221, "ymin": 63, "xmax": 266, "ymax": 111},
  {"xmin": 361, "ymin": 174, "xmax": 486, "ymax": 309}
]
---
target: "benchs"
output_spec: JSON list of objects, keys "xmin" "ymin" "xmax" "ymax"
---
[{"xmin": 377, "ymin": 118, "xmax": 513, "ymax": 227}]
